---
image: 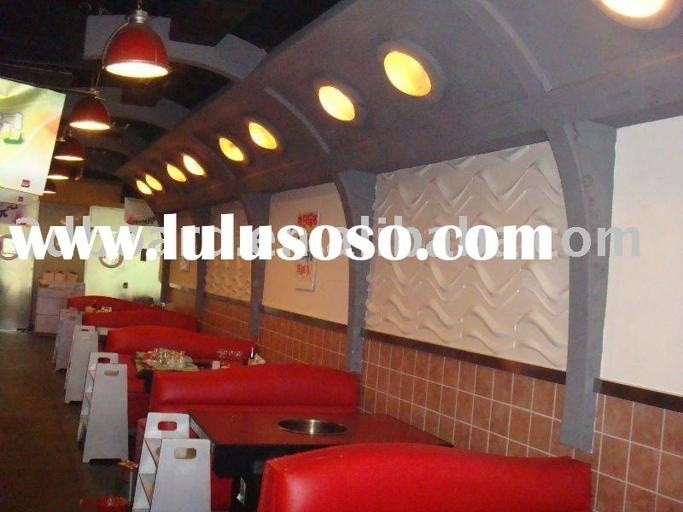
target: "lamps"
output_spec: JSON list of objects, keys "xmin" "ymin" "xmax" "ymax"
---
[
  {"xmin": 68, "ymin": 84, "xmax": 112, "ymax": 131},
  {"xmin": 592, "ymin": 0, "xmax": 683, "ymax": 32},
  {"xmin": 310, "ymin": 77, "xmax": 368, "ymax": 129},
  {"xmin": 99, "ymin": 2, "xmax": 173, "ymax": 78},
  {"xmin": 378, "ymin": 40, "xmax": 447, "ymax": 109},
  {"xmin": 135, "ymin": 115, "xmax": 283, "ymax": 197},
  {"xmin": 41, "ymin": 131, "xmax": 87, "ymax": 195}
]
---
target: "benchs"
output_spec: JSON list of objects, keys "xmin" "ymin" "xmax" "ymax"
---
[{"xmin": 66, "ymin": 294, "xmax": 591, "ymax": 511}]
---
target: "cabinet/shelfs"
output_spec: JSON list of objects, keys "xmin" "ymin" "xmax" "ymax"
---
[{"xmin": 32, "ymin": 286, "xmax": 84, "ymax": 337}]
